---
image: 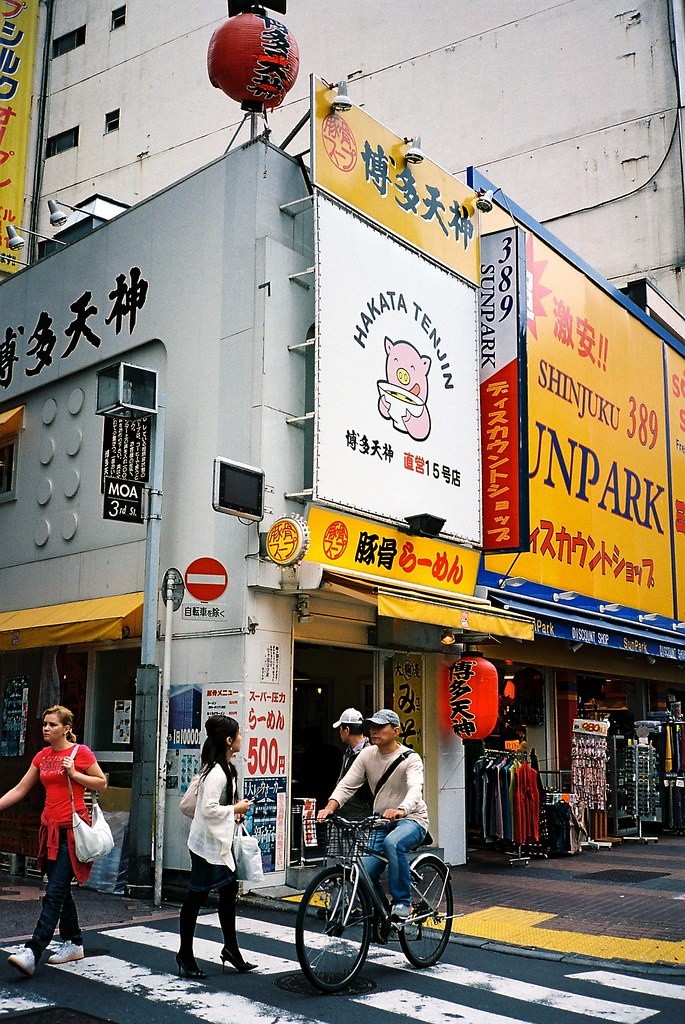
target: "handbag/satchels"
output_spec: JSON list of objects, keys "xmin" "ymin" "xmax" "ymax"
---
[
  {"xmin": 179, "ymin": 772, "xmax": 200, "ymax": 820},
  {"xmin": 232, "ymin": 818, "xmax": 265, "ymax": 883},
  {"xmin": 72, "ymin": 802, "xmax": 115, "ymax": 864}
]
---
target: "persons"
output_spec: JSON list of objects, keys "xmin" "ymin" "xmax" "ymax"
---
[
  {"xmin": 316, "ymin": 709, "xmax": 429, "ymax": 943},
  {"xmin": 176, "ymin": 717, "xmax": 258, "ymax": 978},
  {"xmin": 318, "ymin": 711, "xmax": 374, "ymax": 919},
  {"xmin": 0, "ymin": 704, "xmax": 107, "ymax": 974}
]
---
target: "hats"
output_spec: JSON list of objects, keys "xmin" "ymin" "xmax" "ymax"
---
[
  {"xmin": 365, "ymin": 709, "xmax": 401, "ymax": 727},
  {"xmin": 332, "ymin": 708, "xmax": 364, "ymax": 729}
]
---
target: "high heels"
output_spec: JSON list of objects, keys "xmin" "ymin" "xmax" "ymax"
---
[
  {"xmin": 220, "ymin": 946, "xmax": 259, "ymax": 972},
  {"xmin": 176, "ymin": 950, "xmax": 211, "ymax": 979}
]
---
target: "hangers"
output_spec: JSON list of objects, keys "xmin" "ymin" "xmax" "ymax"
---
[{"xmin": 474, "ymin": 747, "xmax": 539, "ymax": 775}]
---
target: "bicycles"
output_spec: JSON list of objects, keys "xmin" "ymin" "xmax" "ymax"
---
[{"xmin": 296, "ymin": 807, "xmax": 456, "ymax": 993}]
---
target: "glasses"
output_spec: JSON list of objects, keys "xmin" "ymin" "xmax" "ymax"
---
[{"xmin": 626, "ymin": 745, "xmax": 656, "ymax": 816}]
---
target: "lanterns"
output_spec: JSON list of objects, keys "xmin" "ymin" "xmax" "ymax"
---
[{"xmin": 448, "ymin": 651, "xmax": 499, "ymax": 747}]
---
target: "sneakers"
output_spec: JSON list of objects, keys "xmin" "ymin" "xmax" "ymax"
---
[
  {"xmin": 8, "ymin": 947, "xmax": 35, "ymax": 976},
  {"xmin": 390, "ymin": 903, "xmax": 413, "ymax": 921},
  {"xmin": 47, "ymin": 939, "xmax": 84, "ymax": 963}
]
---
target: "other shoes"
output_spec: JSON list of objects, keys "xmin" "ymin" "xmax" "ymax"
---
[
  {"xmin": 343, "ymin": 907, "xmax": 364, "ymax": 919},
  {"xmin": 316, "ymin": 907, "xmax": 345, "ymax": 918}
]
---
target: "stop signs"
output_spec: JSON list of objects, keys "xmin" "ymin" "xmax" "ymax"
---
[{"xmin": 185, "ymin": 557, "xmax": 228, "ymax": 600}]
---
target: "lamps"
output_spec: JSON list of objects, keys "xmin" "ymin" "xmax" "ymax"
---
[
  {"xmin": 46, "ymin": 199, "xmax": 109, "ymax": 227},
  {"xmin": 571, "ymin": 643, "xmax": 585, "ymax": 653},
  {"xmin": 646, "ymin": 655, "xmax": 656, "ymax": 665},
  {"xmin": 598, "ymin": 604, "xmax": 621, "ymax": 613},
  {"xmin": 6, "ymin": 225, "xmax": 67, "ymax": 251},
  {"xmin": 474, "ymin": 188, "xmax": 494, "ymax": 211},
  {"xmin": 673, "ymin": 623, "xmax": 685, "ymax": 630},
  {"xmin": 438, "ymin": 631, "xmax": 456, "ymax": 644},
  {"xmin": 639, "ymin": 613, "xmax": 660, "ymax": 622},
  {"xmin": 331, "ymin": 81, "xmax": 351, "ymax": 111},
  {"xmin": 402, "ymin": 137, "xmax": 424, "ymax": 163},
  {"xmin": 553, "ymin": 591, "xmax": 579, "ymax": 601},
  {"xmin": 498, "ymin": 576, "xmax": 528, "ymax": 588},
  {"xmin": 403, "ymin": 512, "xmax": 447, "ymax": 534}
]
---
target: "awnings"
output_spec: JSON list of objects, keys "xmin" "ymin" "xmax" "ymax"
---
[
  {"xmin": 0, "ymin": 592, "xmax": 145, "ymax": 651},
  {"xmin": 323, "ymin": 571, "xmax": 685, "ymax": 661}
]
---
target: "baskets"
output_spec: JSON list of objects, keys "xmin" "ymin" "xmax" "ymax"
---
[{"xmin": 316, "ymin": 816, "xmax": 378, "ymax": 857}]
